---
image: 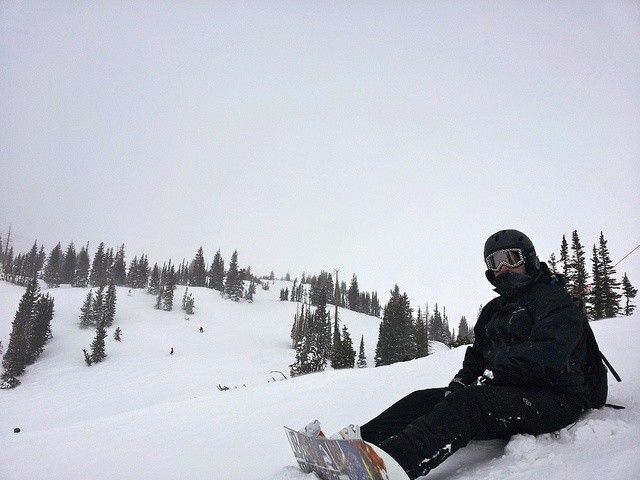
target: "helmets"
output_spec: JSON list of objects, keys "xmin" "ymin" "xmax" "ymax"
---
[{"xmin": 485, "ymin": 229, "xmax": 540, "ymax": 289}]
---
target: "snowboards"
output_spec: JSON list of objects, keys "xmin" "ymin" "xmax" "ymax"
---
[{"xmin": 282, "ymin": 420, "xmax": 410, "ymax": 480}]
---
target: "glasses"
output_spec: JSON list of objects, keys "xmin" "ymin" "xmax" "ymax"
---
[{"xmin": 485, "ymin": 247, "xmax": 535, "ymax": 272}]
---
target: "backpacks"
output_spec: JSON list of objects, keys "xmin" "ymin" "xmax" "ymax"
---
[{"xmin": 487, "ymin": 279, "xmax": 626, "ymax": 410}]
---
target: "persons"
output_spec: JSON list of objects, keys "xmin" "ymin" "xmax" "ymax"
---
[{"xmin": 300, "ymin": 230, "xmax": 589, "ymax": 479}]
